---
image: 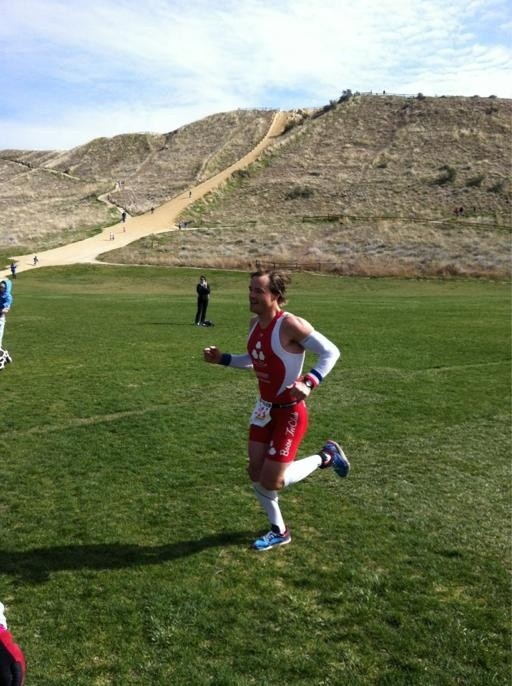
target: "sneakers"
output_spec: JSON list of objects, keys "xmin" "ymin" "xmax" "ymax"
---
[
  {"xmin": 254, "ymin": 530, "xmax": 292, "ymax": 552},
  {"xmin": 0, "ymin": 348, "xmax": 12, "ymax": 370},
  {"xmin": 323, "ymin": 438, "xmax": 351, "ymax": 478}
]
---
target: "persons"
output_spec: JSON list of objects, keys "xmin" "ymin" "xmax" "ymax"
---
[
  {"xmin": 150, "ymin": 207, "xmax": 154, "ymax": 213},
  {"xmin": 122, "ymin": 211, "xmax": 126, "ymax": 223},
  {"xmin": 11, "ymin": 262, "xmax": 16, "ymax": 279},
  {"xmin": 0, "ymin": 279, "xmax": 13, "ymax": 348},
  {"xmin": 202, "ymin": 269, "xmax": 351, "ymax": 551},
  {"xmin": 189, "ymin": 191, "xmax": 191, "ymax": 198},
  {"xmin": 33, "ymin": 256, "xmax": 38, "ymax": 264},
  {"xmin": 195, "ymin": 275, "xmax": 210, "ymax": 326}
]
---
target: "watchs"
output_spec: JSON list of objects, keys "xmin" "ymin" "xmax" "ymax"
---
[{"xmin": 303, "ymin": 377, "xmax": 314, "ymax": 389}]
